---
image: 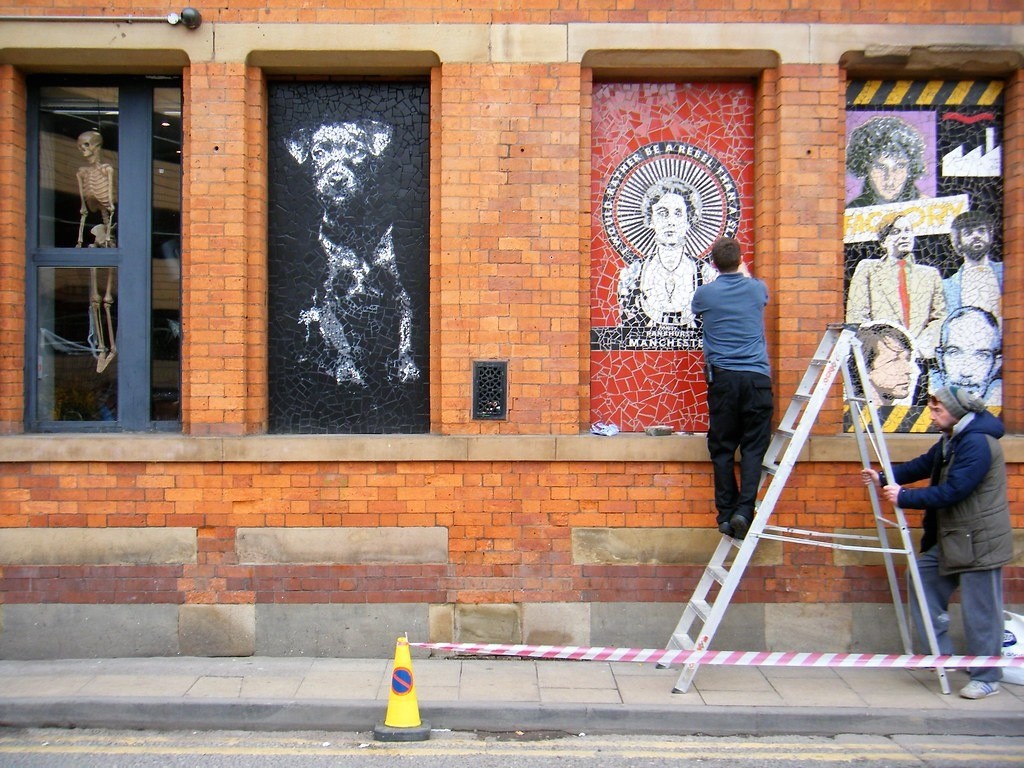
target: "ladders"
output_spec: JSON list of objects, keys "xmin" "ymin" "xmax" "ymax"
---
[{"xmin": 656, "ymin": 323, "xmax": 952, "ymax": 695}]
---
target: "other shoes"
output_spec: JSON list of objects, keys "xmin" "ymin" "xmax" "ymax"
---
[
  {"xmin": 718, "ymin": 522, "xmax": 734, "ymax": 538},
  {"xmin": 730, "ymin": 514, "xmax": 749, "ymax": 539}
]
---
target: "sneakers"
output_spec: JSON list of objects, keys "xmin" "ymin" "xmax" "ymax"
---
[
  {"xmin": 905, "ymin": 654, "xmax": 957, "ymax": 672},
  {"xmin": 959, "ymin": 680, "xmax": 1000, "ymax": 699}
]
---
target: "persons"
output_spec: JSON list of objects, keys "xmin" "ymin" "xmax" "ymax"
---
[
  {"xmin": 691, "ymin": 237, "xmax": 774, "ymax": 539},
  {"xmin": 862, "ymin": 386, "xmax": 1014, "ymax": 699}
]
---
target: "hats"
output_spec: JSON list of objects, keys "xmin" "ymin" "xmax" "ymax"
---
[{"xmin": 936, "ymin": 386, "xmax": 988, "ymax": 420}]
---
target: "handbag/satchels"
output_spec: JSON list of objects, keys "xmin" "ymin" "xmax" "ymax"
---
[{"xmin": 1000, "ymin": 609, "xmax": 1024, "ymax": 685}]
